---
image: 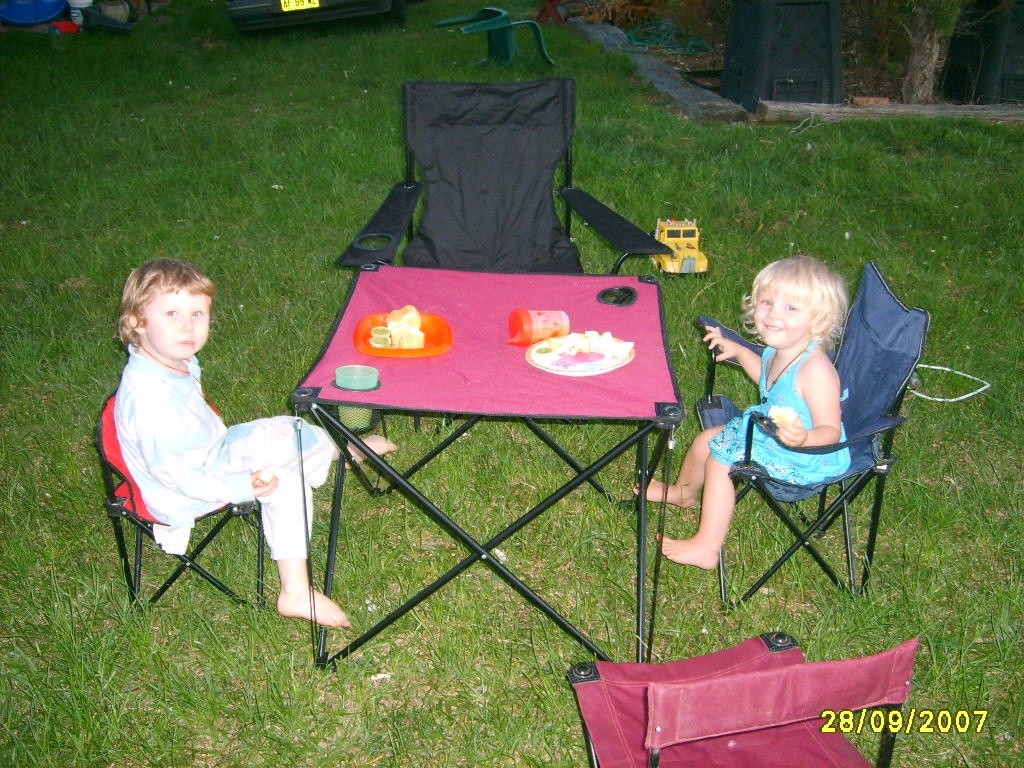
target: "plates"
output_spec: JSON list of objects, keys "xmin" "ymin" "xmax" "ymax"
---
[
  {"xmin": 354, "ymin": 312, "xmax": 453, "ymax": 357},
  {"xmin": 525, "ymin": 334, "xmax": 636, "ymax": 377}
]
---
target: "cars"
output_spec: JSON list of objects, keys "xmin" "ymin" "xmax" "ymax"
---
[{"xmin": 223, "ymin": 0, "xmax": 407, "ymax": 32}]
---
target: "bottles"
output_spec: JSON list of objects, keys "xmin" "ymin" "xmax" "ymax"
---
[{"xmin": 507, "ymin": 310, "xmax": 570, "ymax": 347}]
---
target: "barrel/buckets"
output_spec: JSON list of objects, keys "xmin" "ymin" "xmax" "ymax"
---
[{"xmin": 68, "ymin": 0, "xmax": 93, "ymax": 24}]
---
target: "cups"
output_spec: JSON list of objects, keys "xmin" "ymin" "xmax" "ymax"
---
[{"xmin": 335, "ymin": 365, "xmax": 379, "ymax": 429}]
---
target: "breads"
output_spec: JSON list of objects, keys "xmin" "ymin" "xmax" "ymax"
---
[{"xmin": 548, "ymin": 330, "xmax": 615, "ymax": 354}]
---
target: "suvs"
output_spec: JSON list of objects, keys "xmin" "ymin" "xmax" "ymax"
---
[{"xmin": 655, "ymin": 217, "xmax": 709, "ymax": 275}]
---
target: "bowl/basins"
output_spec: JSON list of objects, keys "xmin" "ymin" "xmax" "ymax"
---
[{"xmin": 0, "ymin": 0, "xmax": 66, "ymax": 25}]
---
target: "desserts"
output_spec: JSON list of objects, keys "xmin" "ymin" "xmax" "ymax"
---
[{"xmin": 370, "ymin": 305, "xmax": 424, "ymax": 349}]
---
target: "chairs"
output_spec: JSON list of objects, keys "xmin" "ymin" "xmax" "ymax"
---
[
  {"xmin": 88, "ymin": 388, "xmax": 267, "ymax": 612},
  {"xmin": 697, "ymin": 256, "xmax": 932, "ymax": 618},
  {"xmin": 335, "ymin": 76, "xmax": 675, "ymax": 437},
  {"xmin": 565, "ymin": 629, "xmax": 919, "ymax": 768},
  {"xmin": 434, "ymin": 4, "xmax": 560, "ymax": 73}
]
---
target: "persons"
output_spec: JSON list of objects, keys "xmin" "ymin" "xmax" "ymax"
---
[
  {"xmin": 113, "ymin": 259, "xmax": 398, "ymax": 627},
  {"xmin": 633, "ymin": 256, "xmax": 851, "ymax": 570}
]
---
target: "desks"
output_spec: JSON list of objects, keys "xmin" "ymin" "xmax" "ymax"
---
[{"xmin": 288, "ymin": 261, "xmax": 686, "ymax": 674}]
